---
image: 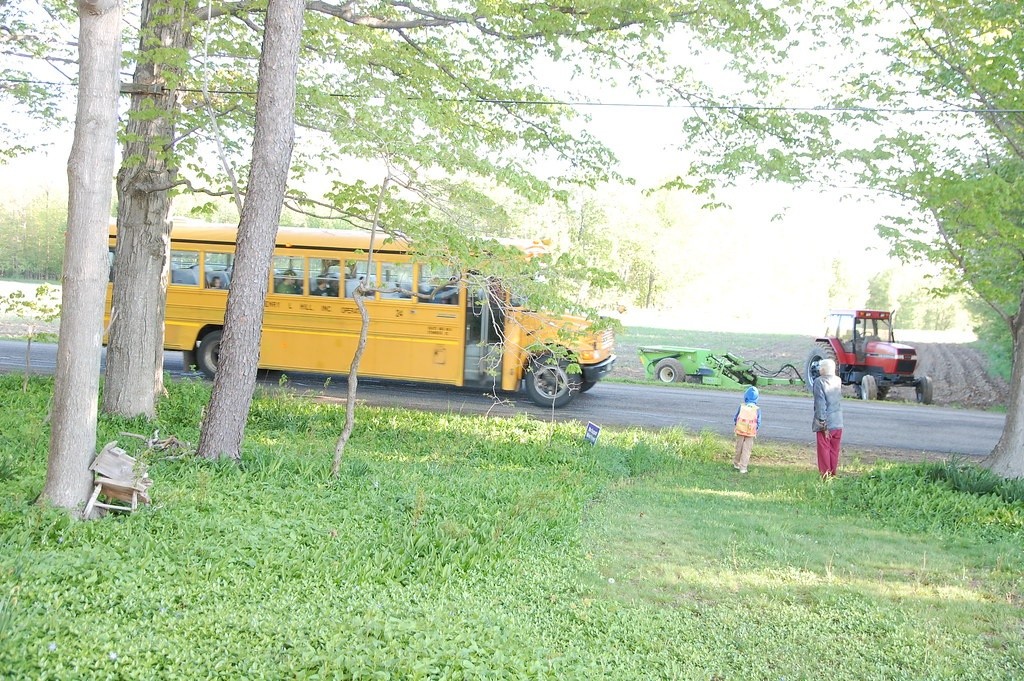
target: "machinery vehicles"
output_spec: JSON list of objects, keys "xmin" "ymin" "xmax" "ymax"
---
[{"xmin": 805, "ymin": 310, "xmax": 934, "ymax": 406}]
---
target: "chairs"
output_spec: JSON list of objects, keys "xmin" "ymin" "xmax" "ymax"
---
[
  {"xmin": 190, "ymin": 264, "xmax": 212, "ymax": 285},
  {"xmin": 170, "ymin": 261, "xmax": 180, "ymax": 270},
  {"xmin": 204, "ymin": 271, "xmax": 232, "ymax": 290},
  {"xmin": 226, "ymin": 266, "xmax": 233, "ymax": 282},
  {"xmin": 172, "ymin": 269, "xmax": 196, "ymax": 286},
  {"xmin": 272, "ymin": 271, "xmax": 452, "ymax": 304}
]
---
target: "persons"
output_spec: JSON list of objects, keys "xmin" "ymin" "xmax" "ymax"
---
[
  {"xmin": 210, "ymin": 270, "xmax": 448, "ymax": 304},
  {"xmin": 733, "ymin": 386, "xmax": 761, "ymax": 473},
  {"xmin": 812, "ymin": 359, "xmax": 844, "ymax": 474}
]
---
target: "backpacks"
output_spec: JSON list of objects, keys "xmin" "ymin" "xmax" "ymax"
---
[{"xmin": 734, "ymin": 401, "xmax": 759, "ymax": 439}]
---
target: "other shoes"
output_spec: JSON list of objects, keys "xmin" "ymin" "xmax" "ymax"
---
[
  {"xmin": 740, "ymin": 469, "xmax": 748, "ymax": 474},
  {"xmin": 733, "ymin": 463, "xmax": 740, "ymax": 469}
]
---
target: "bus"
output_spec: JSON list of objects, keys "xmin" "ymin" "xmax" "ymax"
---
[{"xmin": 60, "ymin": 214, "xmax": 619, "ymax": 408}]
